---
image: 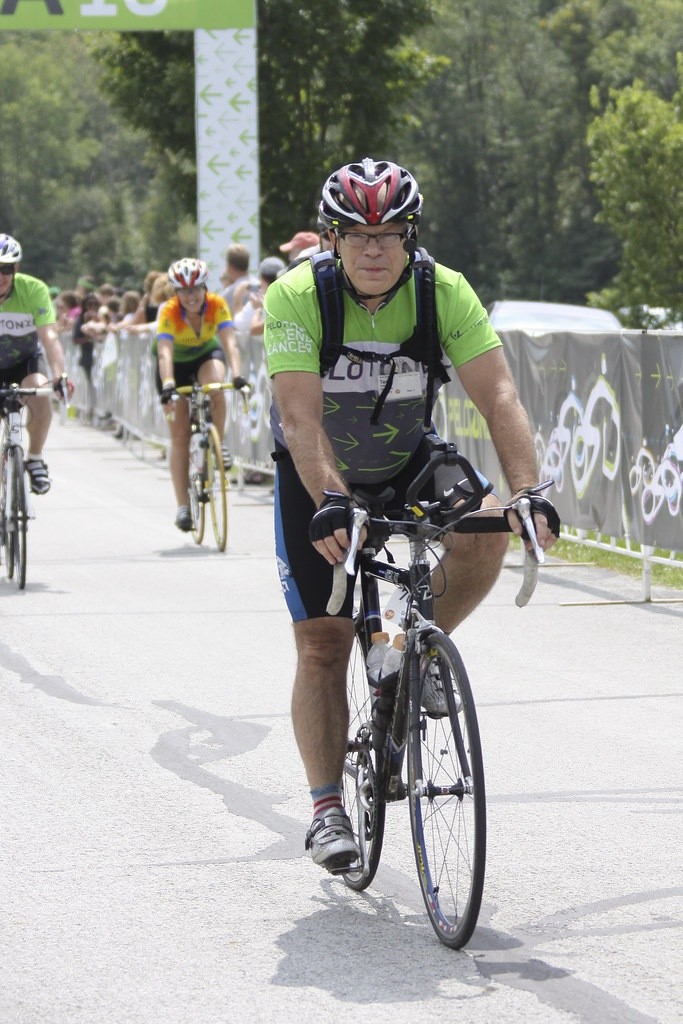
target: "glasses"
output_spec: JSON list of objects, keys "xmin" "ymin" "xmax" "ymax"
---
[
  {"xmin": 338, "ymin": 228, "xmax": 408, "ymax": 248},
  {"xmin": 0, "ymin": 265, "xmax": 14, "ymax": 275}
]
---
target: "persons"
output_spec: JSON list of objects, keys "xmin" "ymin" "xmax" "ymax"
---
[
  {"xmin": 0, "ymin": 233, "xmax": 74, "ymax": 548},
  {"xmin": 52, "ymin": 230, "xmax": 332, "ymax": 485},
  {"xmin": 263, "ymin": 158, "xmax": 560, "ymax": 868},
  {"xmin": 151, "ymin": 255, "xmax": 251, "ymax": 531}
]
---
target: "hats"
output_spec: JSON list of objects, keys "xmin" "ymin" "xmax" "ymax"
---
[
  {"xmin": 279, "ymin": 232, "xmax": 320, "ymax": 251},
  {"xmin": 259, "ymin": 257, "xmax": 285, "ymax": 275}
]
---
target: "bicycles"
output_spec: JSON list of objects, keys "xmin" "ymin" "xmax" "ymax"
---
[
  {"xmin": 164, "ymin": 382, "xmax": 252, "ymax": 553},
  {"xmin": 0, "ymin": 373, "xmax": 71, "ymax": 590},
  {"xmin": 323, "ymin": 480, "xmax": 555, "ymax": 952}
]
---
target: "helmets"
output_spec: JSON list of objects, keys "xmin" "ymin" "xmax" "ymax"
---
[
  {"xmin": 168, "ymin": 258, "xmax": 208, "ymax": 291},
  {"xmin": 0, "ymin": 233, "xmax": 22, "ymax": 263},
  {"xmin": 318, "ymin": 157, "xmax": 424, "ymax": 233}
]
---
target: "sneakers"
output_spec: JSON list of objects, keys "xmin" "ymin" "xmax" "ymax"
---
[
  {"xmin": 304, "ymin": 807, "xmax": 361, "ymax": 875},
  {"xmin": 176, "ymin": 509, "xmax": 193, "ymax": 532},
  {"xmin": 219, "ymin": 447, "xmax": 233, "ymax": 469},
  {"xmin": 423, "ymin": 658, "xmax": 461, "ymax": 715},
  {"xmin": 25, "ymin": 458, "xmax": 50, "ymax": 494}
]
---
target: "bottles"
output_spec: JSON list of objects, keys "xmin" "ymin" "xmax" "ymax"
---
[
  {"xmin": 366, "ymin": 632, "xmax": 391, "ymax": 720},
  {"xmin": 193, "ymin": 428, "xmax": 204, "ymax": 471},
  {"xmin": 380, "ymin": 633, "xmax": 406, "ymax": 679}
]
